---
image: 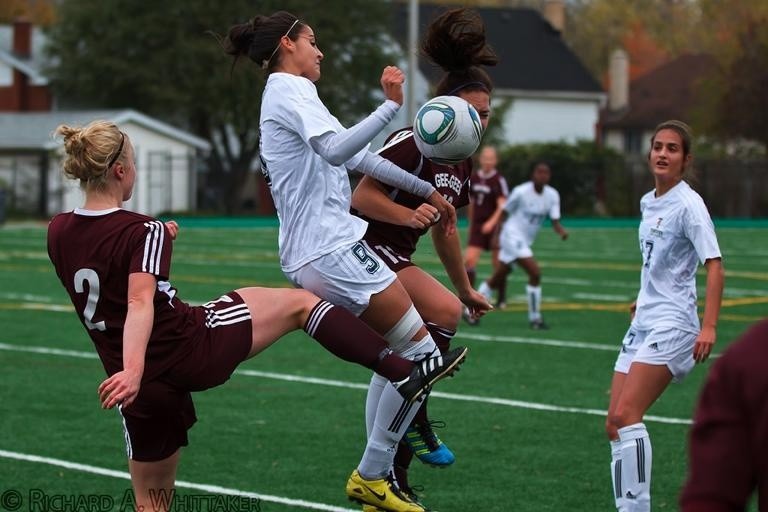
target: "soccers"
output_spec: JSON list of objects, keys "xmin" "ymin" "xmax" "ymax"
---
[{"xmin": 414, "ymin": 96, "xmax": 484, "ymax": 165}]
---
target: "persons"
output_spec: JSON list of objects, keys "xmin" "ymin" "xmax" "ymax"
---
[
  {"xmin": 605, "ymin": 120, "xmax": 726, "ymax": 511},
  {"xmin": 678, "ymin": 313, "xmax": 768, "ymax": 511},
  {"xmin": 457, "ymin": 144, "xmax": 515, "ymax": 325},
  {"xmin": 348, "ymin": 7, "xmax": 493, "ymax": 500},
  {"xmin": 462, "ymin": 158, "xmax": 569, "ymax": 330},
  {"xmin": 46, "ymin": 121, "xmax": 467, "ymax": 511},
  {"xmin": 226, "ymin": 13, "xmax": 458, "ymax": 511}
]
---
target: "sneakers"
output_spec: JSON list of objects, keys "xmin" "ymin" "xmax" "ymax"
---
[
  {"xmin": 389, "ymin": 343, "xmax": 469, "ymax": 402},
  {"xmin": 346, "ymin": 420, "xmax": 455, "ymax": 512}
]
